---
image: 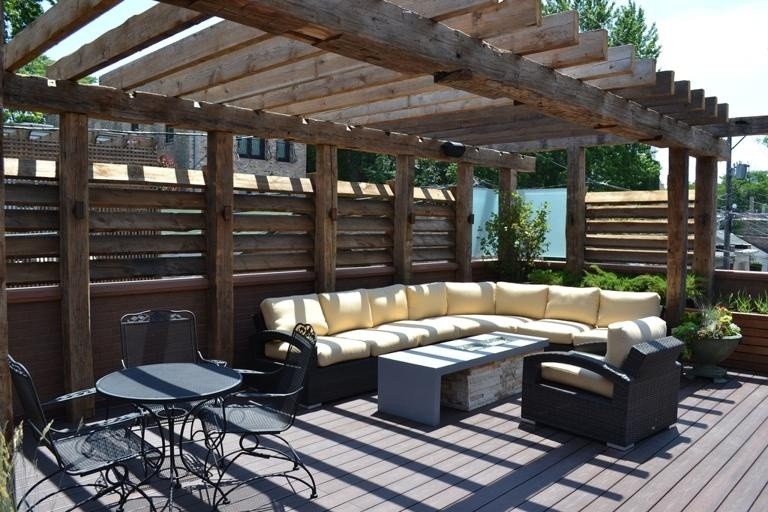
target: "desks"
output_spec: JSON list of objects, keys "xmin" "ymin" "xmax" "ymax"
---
[{"xmin": 96, "ymin": 358, "xmax": 245, "ymax": 511}]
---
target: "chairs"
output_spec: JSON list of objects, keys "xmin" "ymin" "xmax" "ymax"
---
[
  {"xmin": 521, "ymin": 314, "xmax": 683, "ymax": 452},
  {"xmin": 111, "ymin": 308, "xmax": 232, "ymax": 475},
  {"xmin": 5, "ymin": 352, "xmax": 156, "ymax": 510},
  {"xmin": 201, "ymin": 320, "xmax": 319, "ymax": 504}
]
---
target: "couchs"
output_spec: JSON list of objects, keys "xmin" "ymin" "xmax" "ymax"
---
[{"xmin": 253, "ymin": 281, "xmax": 665, "ymax": 410}]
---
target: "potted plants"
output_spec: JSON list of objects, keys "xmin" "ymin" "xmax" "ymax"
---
[{"xmin": 670, "ymin": 293, "xmax": 744, "ymax": 378}]
